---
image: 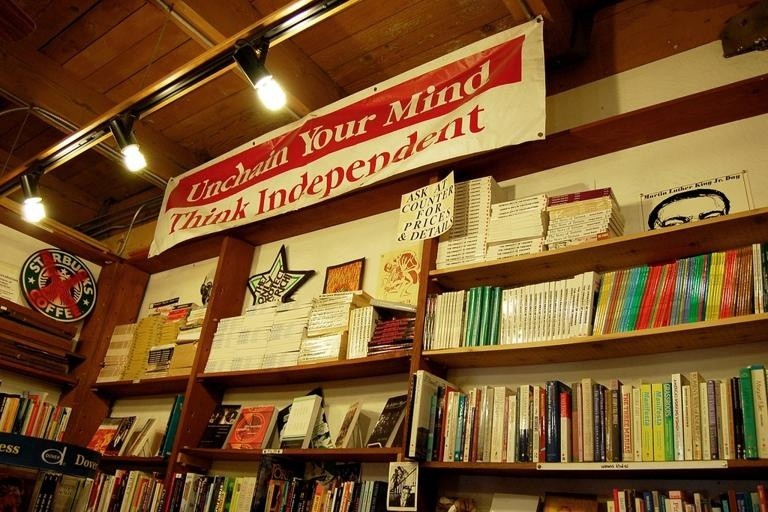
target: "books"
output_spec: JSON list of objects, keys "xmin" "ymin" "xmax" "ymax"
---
[
  {"xmin": 196, "ymin": 386, "xmax": 408, "ymax": 449},
  {"xmin": 424, "ymin": 243, "xmax": 768, "ymax": 352},
  {"xmin": 403, "ymin": 365, "xmax": 768, "ymax": 464},
  {"xmin": 435, "ymin": 177, "xmax": 627, "ymax": 269},
  {"xmin": 3, "ymin": 455, "xmax": 384, "ymax": 512},
  {"xmin": 205, "ymin": 290, "xmax": 417, "ymax": 374},
  {"xmin": 2, "ymin": 392, "xmax": 185, "ymax": 458},
  {"xmin": 96, "ymin": 297, "xmax": 208, "ymax": 383},
  {"xmin": 489, "ymin": 485, "xmax": 766, "ymax": 512}
]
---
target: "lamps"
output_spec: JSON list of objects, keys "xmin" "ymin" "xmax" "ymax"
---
[{"xmin": 18, "ymin": 31, "xmax": 287, "ymax": 225}]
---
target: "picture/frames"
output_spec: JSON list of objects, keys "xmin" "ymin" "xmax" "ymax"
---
[{"xmin": 323, "ymin": 258, "xmax": 365, "ymax": 294}]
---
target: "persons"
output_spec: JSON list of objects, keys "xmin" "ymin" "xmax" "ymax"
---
[{"xmin": 390, "ymin": 486, "xmax": 415, "ymax": 506}]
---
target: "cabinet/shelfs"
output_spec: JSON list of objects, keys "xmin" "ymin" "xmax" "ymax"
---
[
  {"xmin": 71, "ymin": 348, "xmax": 410, "ymax": 467},
  {"xmin": 405, "ymin": 207, "xmax": 766, "ymax": 469}
]
